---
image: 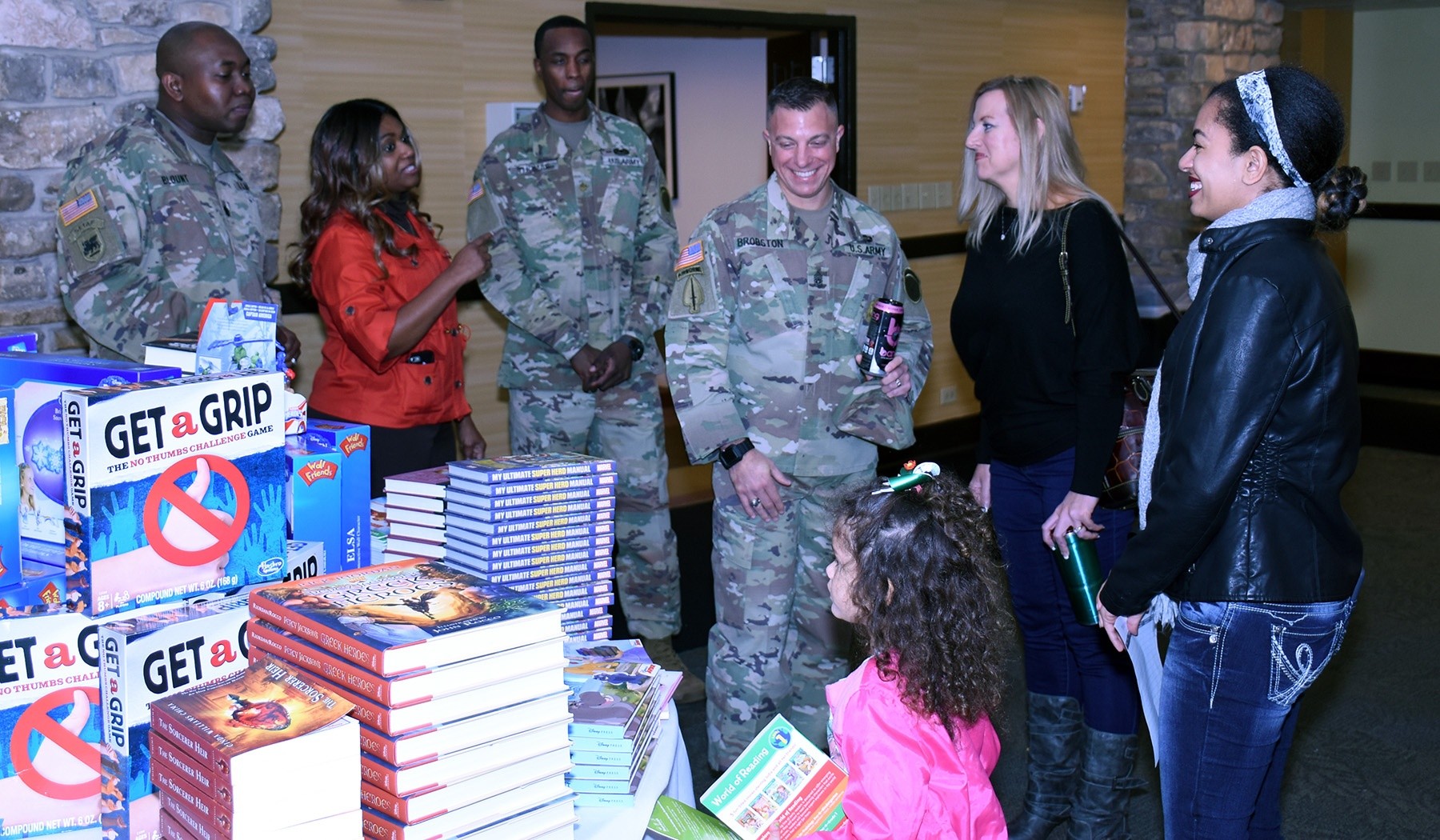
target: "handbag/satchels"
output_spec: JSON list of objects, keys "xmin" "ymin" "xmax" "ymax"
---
[{"xmin": 1103, "ymin": 368, "xmax": 1157, "ymax": 509}]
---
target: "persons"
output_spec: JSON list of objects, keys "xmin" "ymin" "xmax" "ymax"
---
[
  {"xmin": 466, "ymin": 14, "xmax": 707, "ymax": 706},
  {"xmin": 290, "ymin": 98, "xmax": 494, "ymax": 498},
  {"xmin": 1097, "ymin": 64, "xmax": 1368, "ymax": 840},
  {"xmin": 951, "ymin": 77, "xmax": 1144, "ymax": 839},
  {"xmin": 665, "ymin": 76, "xmax": 934, "ymax": 777},
  {"xmin": 52, "ymin": 20, "xmax": 302, "ymax": 374},
  {"xmin": 826, "ymin": 460, "xmax": 1007, "ymax": 840}
]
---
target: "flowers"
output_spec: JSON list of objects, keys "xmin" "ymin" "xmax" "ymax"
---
[{"xmin": 903, "ymin": 460, "xmax": 916, "ymax": 470}]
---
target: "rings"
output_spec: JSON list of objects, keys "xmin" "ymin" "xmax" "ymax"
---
[
  {"xmin": 895, "ymin": 379, "xmax": 903, "ymax": 387},
  {"xmin": 750, "ymin": 497, "xmax": 760, "ymax": 507},
  {"xmin": 291, "ymin": 358, "xmax": 297, "ymax": 365},
  {"xmin": 1073, "ymin": 525, "xmax": 1082, "ymax": 534}
]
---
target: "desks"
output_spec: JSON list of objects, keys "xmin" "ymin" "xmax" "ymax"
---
[{"xmin": 576, "ymin": 698, "xmax": 696, "ymax": 840}]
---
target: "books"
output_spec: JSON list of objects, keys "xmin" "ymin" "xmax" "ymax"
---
[{"xmin": 0, "ymin": 304, "xmax": 847, "ymax": 840}]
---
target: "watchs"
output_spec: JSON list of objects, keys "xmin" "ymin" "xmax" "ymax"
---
[{"xmin": 718, "ymin": 437, "xmax": 755, "ymax": 469}]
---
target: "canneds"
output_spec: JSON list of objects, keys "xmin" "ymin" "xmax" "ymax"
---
[{"xmin": 859, "ymin": 297, "xmax": 903, "ymax": 377}]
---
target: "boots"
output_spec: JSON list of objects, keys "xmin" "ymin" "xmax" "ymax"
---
[
  {"xmin": 1071, "ymin": 722, "xmax": 1140, "ymax": 840},
  {"xmin": 642, "ymin": 634, "xmax": 706, "ymax": 703},
  {"xmin": 1006, "ymin": 689, "xmax": 1084, "ymax": 840}
]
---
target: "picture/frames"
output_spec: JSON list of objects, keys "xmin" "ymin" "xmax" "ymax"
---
[{"xmin": 596, "ymin": 72, "xmax": 678, "ymax": 200}]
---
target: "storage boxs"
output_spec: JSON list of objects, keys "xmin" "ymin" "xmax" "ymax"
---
[{"xmin": 0, "ymin": 332, "xmax": 372, "ymax": 840}]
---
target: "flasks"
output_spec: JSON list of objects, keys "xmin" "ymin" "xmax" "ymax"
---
[{"xmin": 1051, "ymin": 528, "xmax": 1103, "ymax": 626}]
